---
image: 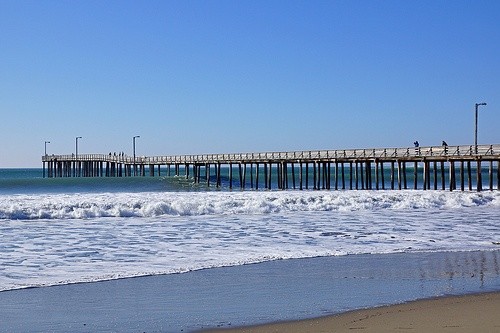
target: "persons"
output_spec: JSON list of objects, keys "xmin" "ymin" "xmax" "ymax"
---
[
  {"xmin": 413, "ymin": 140, "xmax": 420, "ymax": 155},
  {"xmin": 119, "ymin": 151, "xmax": 121, "ymax": 156},
  {"xmin": 442, "ymin": 140, "xmax": 449, "ymax": 155},
  {"xmin": 108, "ymin": 151, "xmax": 112, "ymax": 157},
  {"xmin": 113, "ymin": 151, "xmax": 116, "ymax": 156},
  {"xmin": 122, "ymin": 151, "xmax": 125, "ymax": 156}
]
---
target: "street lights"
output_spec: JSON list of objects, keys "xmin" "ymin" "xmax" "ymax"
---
[
  {"xmin": 76, "ymin": 137, "xmax": 82, "ymax": 160},
  {"xmin": 474, "ymin": 102, "xmax": 487, "ymax": 155},
  {"xmin": 133, "ymin": 135, "xmax": 141, "ymax": 164},
  {"xmin": 45, "ymin": 141, "xmax": 50, "ymax": 156}
]
